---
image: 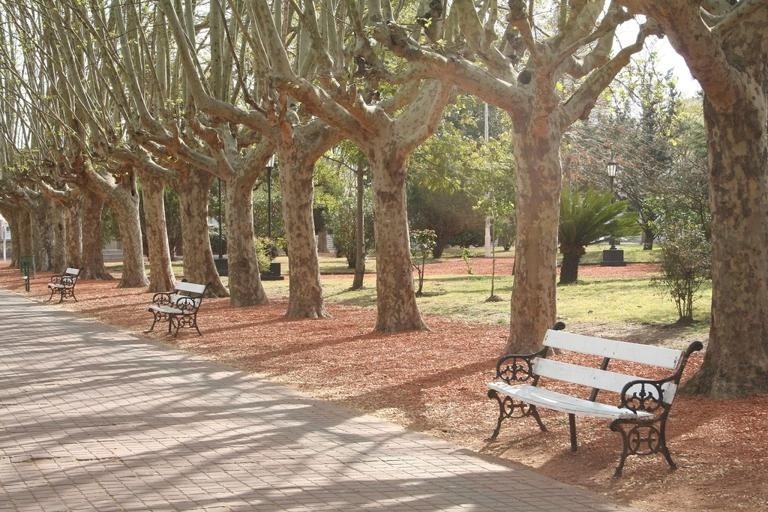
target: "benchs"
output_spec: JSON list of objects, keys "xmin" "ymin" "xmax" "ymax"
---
[
  {"xmin": 487, "ymin": 323, "xmax": 703, "ymax": 479},
  {"xmin": 48, "ymin": 268, "xmax": 82, "ymax": 303},
  {"xmin": 148, "ymin": 279, "xmax": 212, "ymax": 339}
]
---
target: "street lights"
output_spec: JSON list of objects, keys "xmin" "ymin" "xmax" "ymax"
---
[
  {"xmin": 265, "ymin": 154, "xmax": 276, "ymax": 238},
  {"xmin": 606, "ymin": 160, "xmax": 618, "ymax": 249}
]
